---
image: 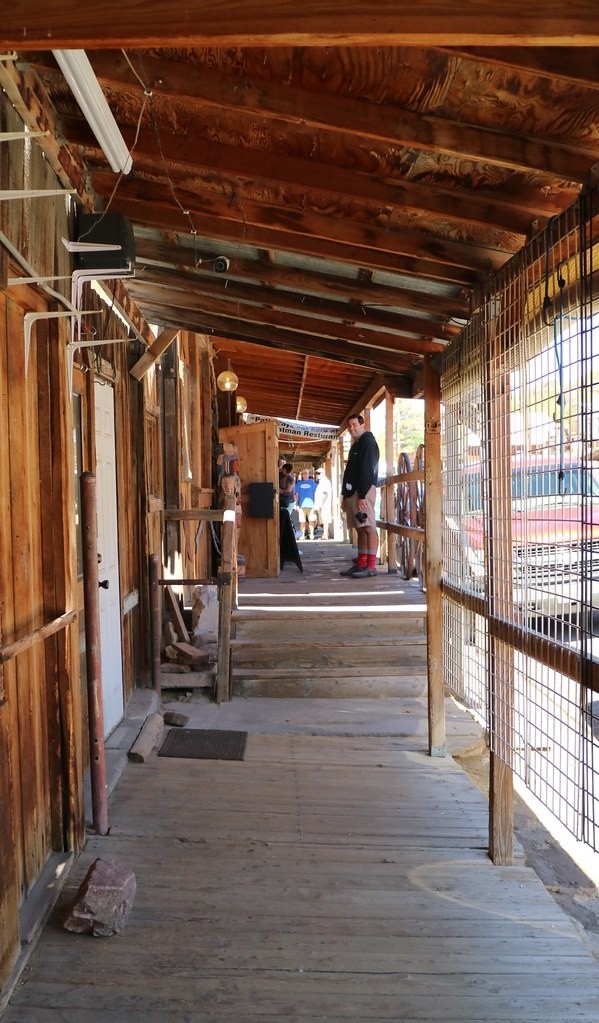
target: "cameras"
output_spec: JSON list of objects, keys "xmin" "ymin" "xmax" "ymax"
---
[{"xmin": 355, "ymin": 511, "xmax": 368, "ymax": 524}]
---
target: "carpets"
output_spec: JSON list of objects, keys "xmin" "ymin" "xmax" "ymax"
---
[{"xmin": 157, "ymin": 728, "xmax": 248, "ymax": 760}]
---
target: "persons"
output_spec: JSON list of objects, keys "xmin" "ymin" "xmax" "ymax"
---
[
  {"xmin": 340, "ymin": 415, "xmax": 380, "ymax": 577},
  {"xmin": 278, "ymin": 459, "xmax": 331, "ymax": 539},
  {"xmin": 216, "ymin": 441, "xmax": 246, "ymax": 562}
]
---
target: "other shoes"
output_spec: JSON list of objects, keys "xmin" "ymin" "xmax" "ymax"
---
[
  {"xmin": 299, "ymin": 536, "xmax": 306, "ymax": 540},
  {"xmin": 310, "ymin": 535, "xmax": 314, "ymax": 540},
  {"xmin": 320, "ymin": 536, "xmax": 328, "ymax": 541}
]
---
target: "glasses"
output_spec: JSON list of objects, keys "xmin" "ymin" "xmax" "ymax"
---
[{"xmin": 316, "ymin": 472, "xmax": 320, "ymax": 475}]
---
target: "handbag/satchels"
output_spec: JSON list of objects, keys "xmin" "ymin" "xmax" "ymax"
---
[{"xmin": 280, "ymin": 495, "xmax": 291, "ymax": 508}]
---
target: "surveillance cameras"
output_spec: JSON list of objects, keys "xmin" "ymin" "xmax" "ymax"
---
[{"xmin": 214, "ymin": 257, "xmax": 230, "ymax": 273}]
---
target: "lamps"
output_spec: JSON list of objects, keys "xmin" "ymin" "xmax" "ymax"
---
[
  {"xmin": 216, "ymin": 358, "xmax": 239, "ymax": 391},
  {"xmin": 52, "ymin": 49, "xmax": 134, "ymax": 175},
  {"xmin": 235, "ymin": 396, "xmax": 247, "ymax": 413}
]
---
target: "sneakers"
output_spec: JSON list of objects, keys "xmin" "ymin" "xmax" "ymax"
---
[
  {"xmin": 339, "ymin": 565, "xmax": 362, "ymax": 576},
  {"xmin": 352, "ymin": 567, "xmax": 377, "ymax": 577}
]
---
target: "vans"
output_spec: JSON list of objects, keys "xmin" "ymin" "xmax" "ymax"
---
[{"xmin": 446, "ymin": 455, "xmax": 599, "ymax": 619}]
